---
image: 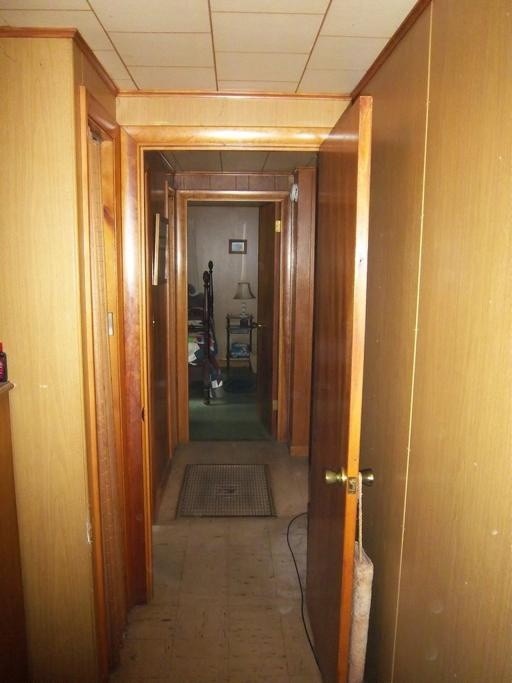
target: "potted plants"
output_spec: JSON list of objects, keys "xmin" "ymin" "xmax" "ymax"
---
[
  {"xmin": 228, "ymin": 238, "xmax": 248, "ymax": 254},
  {"xmin": 151, "ymin": 212, "xmax": 169, "ymax": 287}
]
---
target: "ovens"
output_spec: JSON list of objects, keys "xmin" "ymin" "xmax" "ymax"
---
[{"xmin": 188, "ymin": 259, "xmax": 223, "ymax": 403}]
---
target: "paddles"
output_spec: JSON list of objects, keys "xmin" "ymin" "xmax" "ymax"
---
[{"xmin": 175, "ymin": 464, "xmax": 278, "ymax": 518}]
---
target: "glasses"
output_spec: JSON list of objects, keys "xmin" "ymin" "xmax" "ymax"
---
[{"xmin": 233, "ymin": 280, "xmax": 256, "ymax": 324}]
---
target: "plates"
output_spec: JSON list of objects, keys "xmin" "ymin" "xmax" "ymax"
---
[{"xmin": 226, "ymin": 312, "xmax": 254, "ymax": 378}]
